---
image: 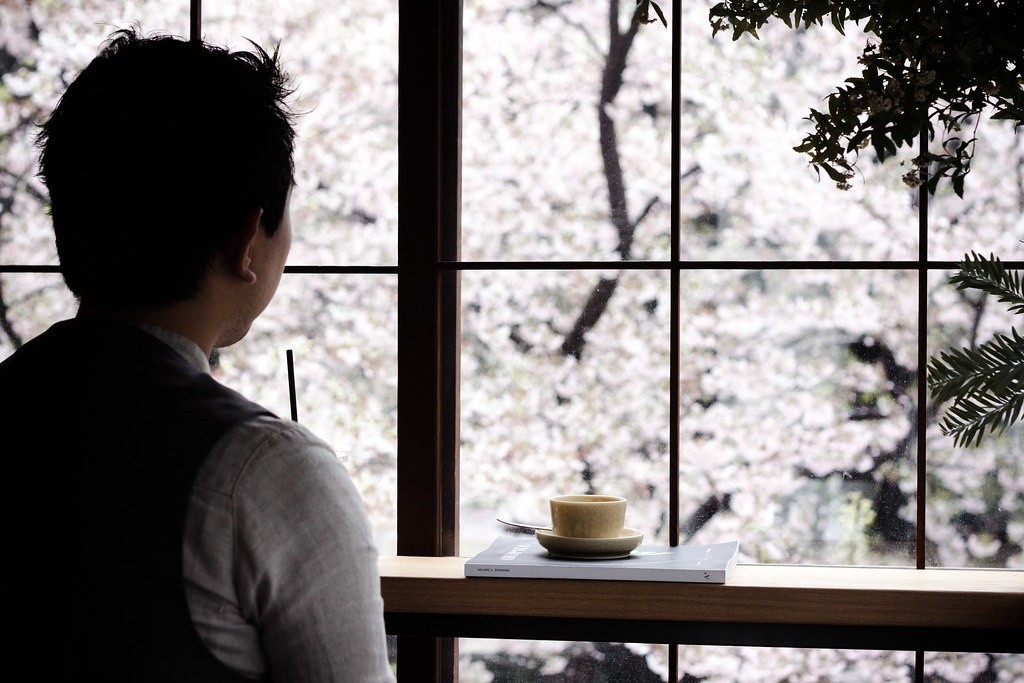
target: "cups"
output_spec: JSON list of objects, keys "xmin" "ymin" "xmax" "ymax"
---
[{"xmin": 550, "ymin": 494, "xmax": 627, "ymax": 539}]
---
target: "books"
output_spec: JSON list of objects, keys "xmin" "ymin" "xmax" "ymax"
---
[{"xmin": 464, "ymin": 536, "xmax": 739, "ymax": 584}]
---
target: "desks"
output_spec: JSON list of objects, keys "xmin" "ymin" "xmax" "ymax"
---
[{"xmin": 376, "ymin": 556, "xmax": 1024, "ymax": 683}]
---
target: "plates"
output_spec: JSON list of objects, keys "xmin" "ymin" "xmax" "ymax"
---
[{"xmin": 535, "ymin": 528, "xmax": 645, "ymax": 560}]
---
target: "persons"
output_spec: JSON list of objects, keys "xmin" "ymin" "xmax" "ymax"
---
[{"xmin": 0, "ymin": 27, "xmax": 397, "ymax": 683}]
